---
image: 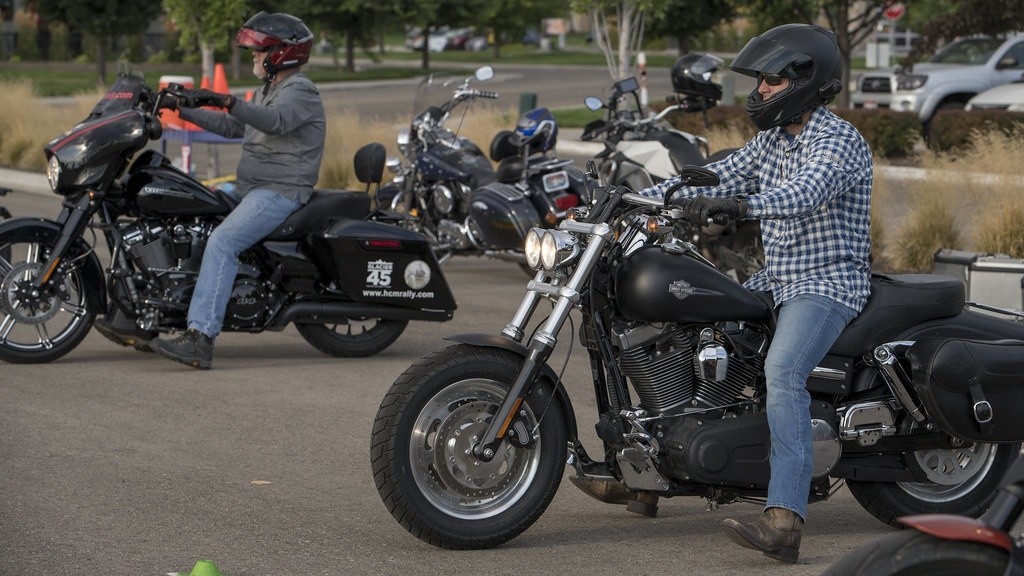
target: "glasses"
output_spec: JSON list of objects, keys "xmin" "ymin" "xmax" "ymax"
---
[{"xmin": 755, "ymin": 72, "xmax": 786, "ymax": 87}]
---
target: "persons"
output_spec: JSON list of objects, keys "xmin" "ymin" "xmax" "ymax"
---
[
  {"xmin": 567, "ymin": 23, "xmax": 875, "ymax": 564},
  {"xmin": 90, "ymin": 10, "xmax": 325, "ymax": 374}
]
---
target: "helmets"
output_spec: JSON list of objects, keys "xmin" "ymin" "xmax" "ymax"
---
[
  {"xmin": 729, "ymin": 23, "xmax": 845, "ymax": 129},
  {"xmin": 672, "ymin": 51, "xmax": 723, "ymax": 99},
  {"xmin": 235, "ymin": 10, "xmax": 314, "ymax": 71},
  {"xmin": 511, "ymin": 106, "xmax": 558, "ymax": 147}
]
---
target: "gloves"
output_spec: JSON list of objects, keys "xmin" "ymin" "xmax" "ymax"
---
[
  {"xmin": 181, "ymin": 89, "xmax": 228, "ymax": 111},
  {"xmin": 142, "ymin": 92, "xmax": 177, "ymax": 113},
  {"xmin": 671, "ymin": 197, "xmax": 738, "ymax": 227}
]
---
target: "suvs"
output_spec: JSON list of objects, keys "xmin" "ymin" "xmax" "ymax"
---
[{"xmin": 851, "ymin": 31, "xmax": 1024, "ymax": 130}]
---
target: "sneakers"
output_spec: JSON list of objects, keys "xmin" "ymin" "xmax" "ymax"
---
[
  {"xmin": 94, "ymin": 317, "xmax": 158, "ymax": 352},
  {"xmin": 150, "ymin": 328, "xmax": 214, "ymax": 370}
]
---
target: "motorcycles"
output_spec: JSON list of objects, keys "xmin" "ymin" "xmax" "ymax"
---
[
  {"xmin": 368, "ymin": 159, "xmax": 1024, "ymax": 550},
  {"xmin": 375, "ymin": 64, "xmax": 602, "ymax": 283},
  {"xmin": 0, "ymin": 65, "xmax": 458, "ymax": 366},
  {"xmin": 816, "ymin": 447, "xmax": 1024, "ymax": 575},
  {"xmin": 580, "ymin": 76, "xmax": 769, "ymax": 289}
]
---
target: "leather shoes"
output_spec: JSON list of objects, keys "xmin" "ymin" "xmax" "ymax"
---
[
  {"xmin": 568, "ymin": 476, "xmax": 658, "ymax": 516},
  {"xmin": 722, "ymin": 507, "xmax": 803, "ymax": 563}
]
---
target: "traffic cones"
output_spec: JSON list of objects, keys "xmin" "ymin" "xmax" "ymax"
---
[
  {"xmin": 182, "ymin": 74, "xmax": 212, "ymax": 131},
  {"xmin": 205, "ymin": 64, "xmax": 231, "ymax": 113}
]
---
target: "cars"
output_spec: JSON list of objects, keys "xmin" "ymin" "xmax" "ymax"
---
[
  {"xmin": 403, "ymin": 24, "xmax": 549, "ymax": 52},
  {"xmin": 963, "ymin": 72, "xmax": 1024, "ymax": 113}
]
